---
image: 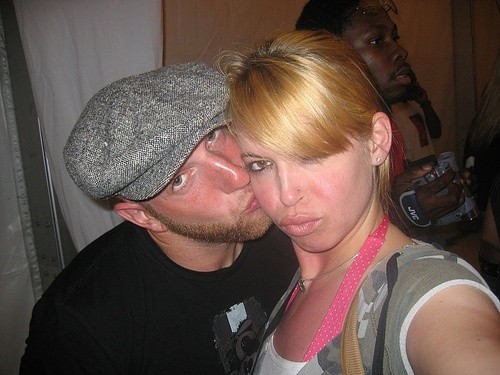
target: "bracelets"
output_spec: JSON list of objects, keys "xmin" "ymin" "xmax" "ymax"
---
[{"xmin": 420, "ymin": 100, "xmax": 432, "ymax": 108}]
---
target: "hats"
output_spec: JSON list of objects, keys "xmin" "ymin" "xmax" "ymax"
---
[{"xmin": 63, "ymin": 62, "xmax": 232, "ymax": 200}]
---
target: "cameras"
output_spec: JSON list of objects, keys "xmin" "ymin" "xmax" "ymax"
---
[{"xmin": 409, "ymin": 151, "xmax": 479, "ymax": 226}]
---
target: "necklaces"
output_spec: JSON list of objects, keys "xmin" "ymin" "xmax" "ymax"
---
[{"xmin": 297, "ymin": 251, "xmax": 360, "ymax": 293}]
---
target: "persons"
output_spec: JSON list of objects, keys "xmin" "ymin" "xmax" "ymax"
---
[
  {"xmin": 219, "ymin": 29, "xmax": 500, "ymax": 375},
  {"xmin": 19, "ymin": 62, "xmax": 300, "ymax": 375},
  {"xmin": 294, "ymin": 0, "xmax": 500, "ymax": 301}
]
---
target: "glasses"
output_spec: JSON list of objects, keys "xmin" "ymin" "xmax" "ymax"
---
[{"xmin": 340, "ymin": 0, "xmax": 398, "ymax": 18}]
